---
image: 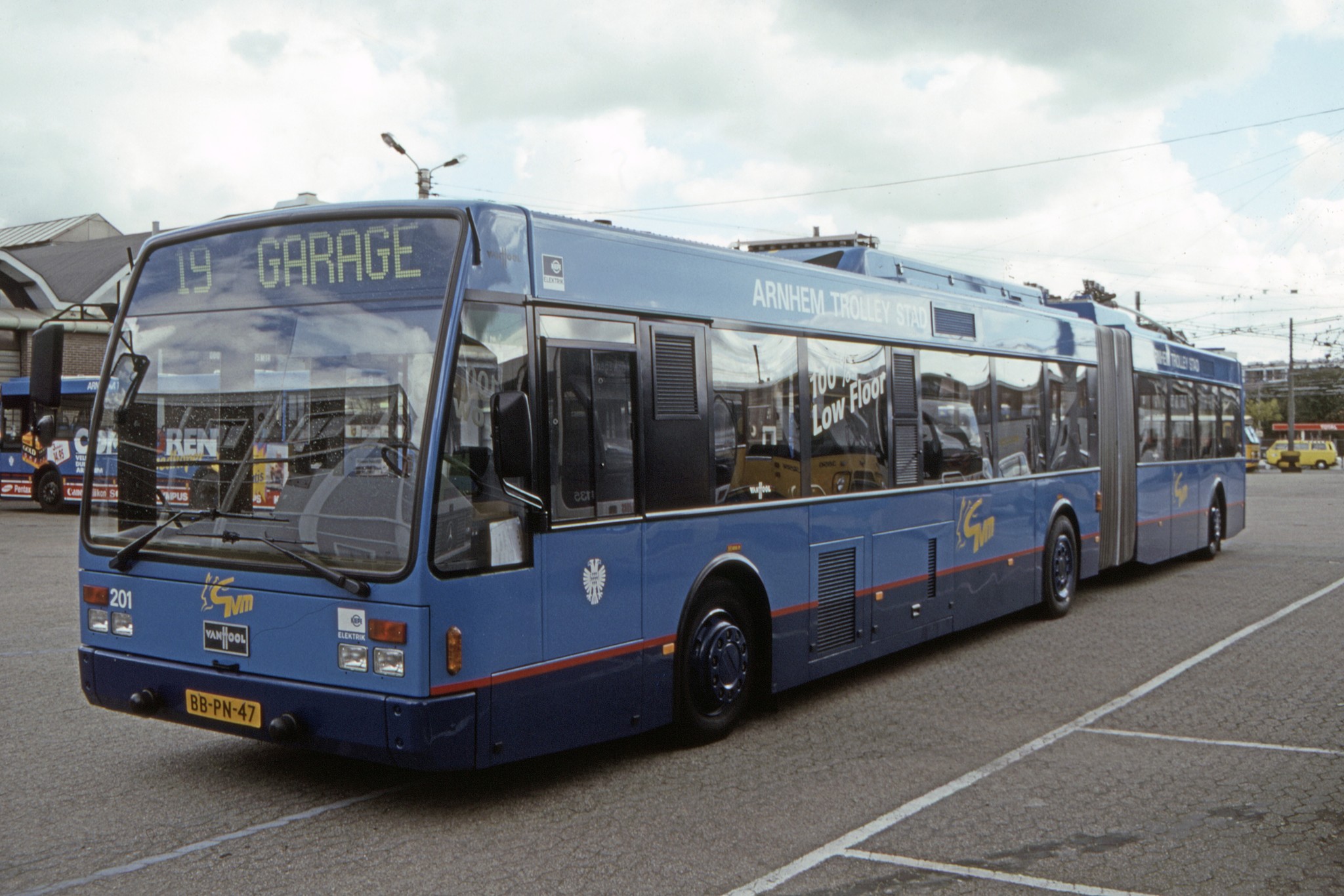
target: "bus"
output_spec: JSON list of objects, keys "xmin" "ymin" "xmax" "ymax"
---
[
  {"xmin": 0, "ymin": 376, "xmax": 292, "ymax": 509},
  {"xmin": 494, "ymin": 339, "xmax": 638, "ymax": 518},
  {"xmin": 1139, "ymin": 416, "xmax": 1261, "ymax": 471},
  {"xmin": 28, "ymin": 196, "xmax": 1241, "ymax": 772},
  {"xmin": 711, "ymin": 371, "xmax": 888, "ymax": 500}
]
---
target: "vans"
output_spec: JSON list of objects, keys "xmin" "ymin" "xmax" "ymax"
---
[
  {"xmin": 1265, "ymin": 440, "xmax": 1342, "ymax": 469},
  {"xmin": 920, "ymin": 396, "xmax": 986, "ymax": 474}
]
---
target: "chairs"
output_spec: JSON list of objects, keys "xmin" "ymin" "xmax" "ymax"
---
[{"xmin": 59, "ymin": 415, "xmax": 78, "ymax": 437}]
